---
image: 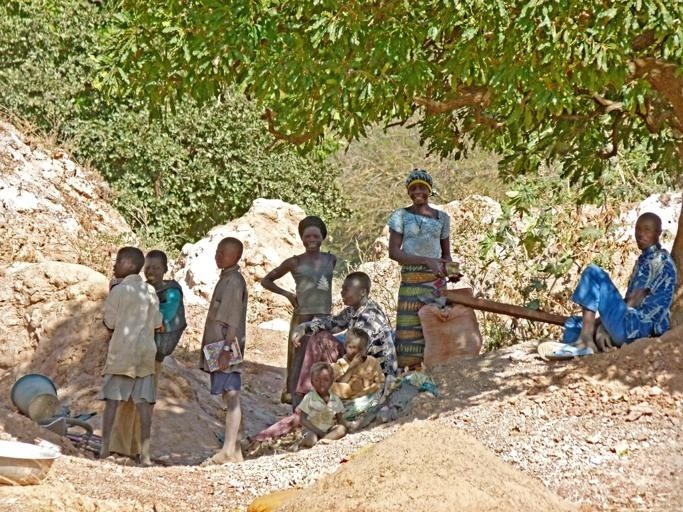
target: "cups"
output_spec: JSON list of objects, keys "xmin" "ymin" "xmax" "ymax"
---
[{"xmin": 444, "ymin": 254, "xmax": 461, "ymax": 279}]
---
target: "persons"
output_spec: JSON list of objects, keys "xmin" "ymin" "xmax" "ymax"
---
[
  {"xmin": 537, "ymin": 212, "xmax": 678, "ymax": 360},
  {"xmin": 108, "ymin": 249, "xmax": 187, "ymax": 463},
  {"xmin": 389, "ymin": 168, "xmax": 463, "ymax": 369},
  {"xmin": 100, "ymin": 246, "xmax": 163, "ymax": 467},
  {"xmin": 262, "ymin": 216, "xmax": 336, "ymax": 403},
  {"xmin": 302, "ymin": 361, "xmax": 348, "ymax": 446},
  {"xmin": 199, "ymin": 236, "xmax": 248, "ymax": 465},
  {"xmin": 327, "ymin": 328, "xmax": 386, "ymax": 433},
  {"xmin": 241, "ymin": 272, "xmax": 398, "ymax": 449}
]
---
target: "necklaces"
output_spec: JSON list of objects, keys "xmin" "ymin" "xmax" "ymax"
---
[{"xmin": 411, "ymin": 204, "xmax": 430, "ymax": 238}]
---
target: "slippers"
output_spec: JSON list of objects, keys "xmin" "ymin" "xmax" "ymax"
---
[{"xmin": 545, "ymin": 345, "xmax": 593, "ymax": 360}]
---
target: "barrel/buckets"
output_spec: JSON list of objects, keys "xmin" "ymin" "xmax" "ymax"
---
[{"xmin": 10, "ymin": 374, "xmax": 61, "ymax": 423}]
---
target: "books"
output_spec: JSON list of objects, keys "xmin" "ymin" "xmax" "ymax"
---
[{"xmin": 203, "ymin": 336, "xmax": 244, "ymax": 373}]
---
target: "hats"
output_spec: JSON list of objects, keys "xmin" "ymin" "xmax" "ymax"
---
[{"xmin": 406, "ymin": 170, "xmax": 432, "ymax": 192}]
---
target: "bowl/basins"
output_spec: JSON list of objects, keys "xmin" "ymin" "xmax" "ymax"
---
[{"xmin": 0, "ymin": 441, "xmax": 61, "ymax": 486}]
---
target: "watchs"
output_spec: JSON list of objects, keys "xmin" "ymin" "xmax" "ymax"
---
[{"xmin": 222, "ymin": 345, "xmax": 233, "ymax": 353}]
